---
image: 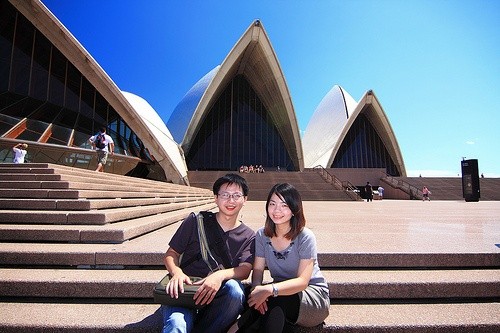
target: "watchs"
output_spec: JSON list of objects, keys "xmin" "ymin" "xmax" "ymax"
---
[{"xmin": 272, "ymin": 283, "xmax": 278, "ymax": 297}]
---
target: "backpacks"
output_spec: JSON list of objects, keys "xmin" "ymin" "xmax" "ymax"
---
[{"xmin": 95, "ymin": 133, "xmax": 106, "ymax": 148}]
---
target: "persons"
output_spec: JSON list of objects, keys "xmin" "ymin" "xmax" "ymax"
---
[
  {"xmin": 240, "ymin": 165, "xmax": 248, "ymax": 173},
  {"xmin": 249, "ymin": 165, "xmax": 254, "ymax": 173},
  {"xmin": 12, "ymin": 143, "xmax": 28, "ymax": 163},
  {"xmin": 409, "ymin": 190, "xmax": 413, "ymax": 200},
  {"xmin": 481, "ymin": 173, "xmax": 484, "ymax": 179},
  {"xmin": 235, "ymin": 182, "xmax": 330, "ymax": 333},
  {"xmin": 152, "ymin": 173, "xmax": 256, "ymax": 333},
  {"xmin": 88, "ymin": 127, "xmax": 114, "ymax": 172},
  {"xmin": 365, "ymin": 181, "xmax": 373, "ymax": 202},
  {"xmin": 378, "ymin": 185, "xmax": 384, "ymax": 200},
  {"xmin": 422, "ymin": 186, "xmax": 430, "ymax": 201},
  {"xmin": 255, "ymin": 164, "xmax": 265, "ymax": 173}
]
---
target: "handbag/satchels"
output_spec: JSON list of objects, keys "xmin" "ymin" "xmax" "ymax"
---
[{"xmin": 153, "ymin": 272, "xmax": 213, "ymax": 309}]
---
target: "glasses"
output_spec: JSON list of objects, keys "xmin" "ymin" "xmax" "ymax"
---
[{"xmin": 217, "ymin": 192, "xmax": 245, "ymax": 200}]
